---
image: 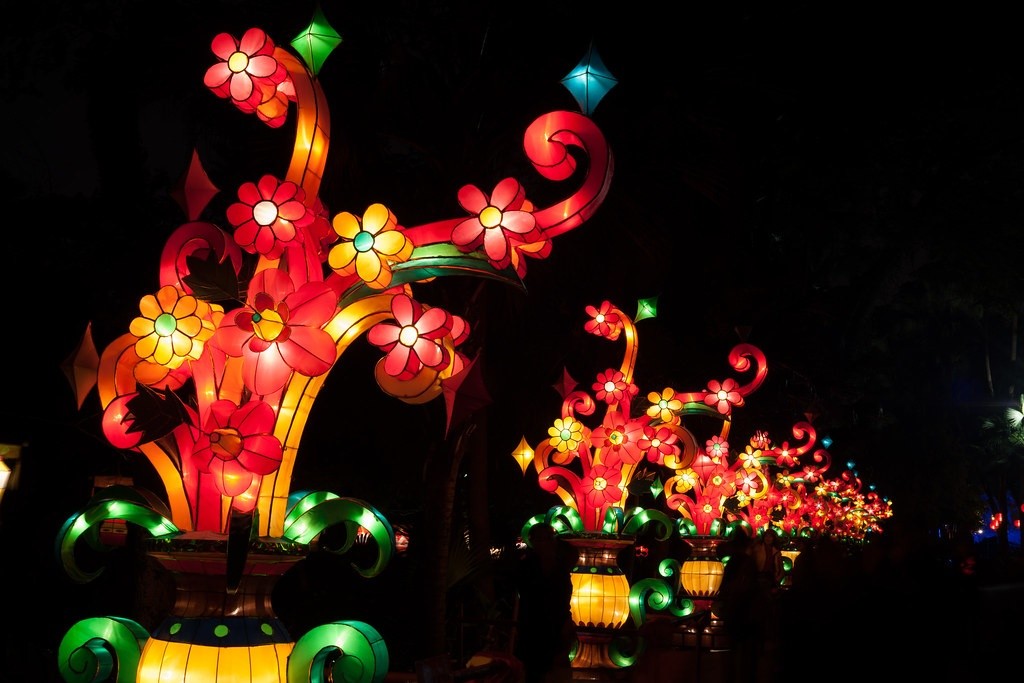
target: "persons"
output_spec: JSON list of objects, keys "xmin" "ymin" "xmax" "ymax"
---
[{"xmin": 0, "ymin": 434, "xmax": 1024, "ymax": 683}]
